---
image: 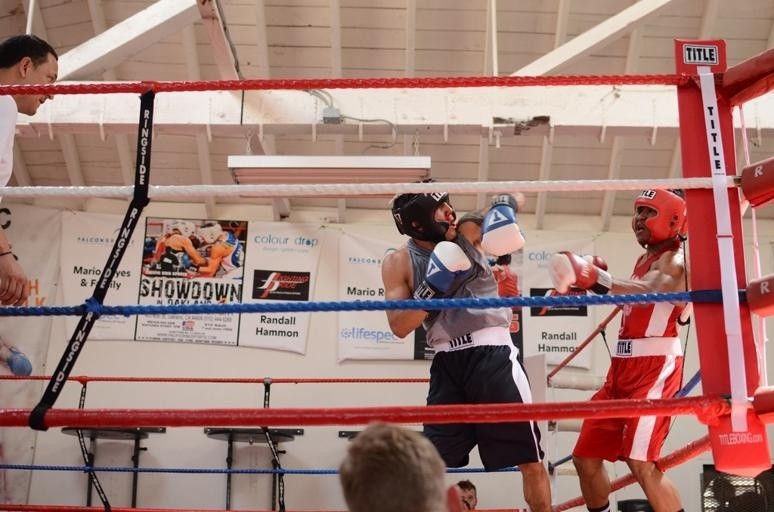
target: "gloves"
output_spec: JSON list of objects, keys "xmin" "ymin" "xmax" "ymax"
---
[
  {"xmin": 412, "ymin": 242, "xmax": 484, "ymax": 311},
  {"xmin": 547, "ymin": 247, "xmax": 614, "ymax": 295},
  {"xmin": 479, "ymin": 192, "xmax": 527, "ymax": 256}
]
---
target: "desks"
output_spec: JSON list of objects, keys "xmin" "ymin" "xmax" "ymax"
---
[{"xmin": 62, "ymin": 426, "xmax": 305, "ymax": 510}]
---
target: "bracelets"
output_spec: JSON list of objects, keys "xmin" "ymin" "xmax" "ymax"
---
[{"xmin": 0, "ymin": 251, "xmax": 12, "ymax": 257}]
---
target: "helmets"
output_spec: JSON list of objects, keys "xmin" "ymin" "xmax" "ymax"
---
[
  {"xmin": 631, "ymin": 188, "xmax": 687, "ymax": 247},
  {"xmin": 388, "ymin": 178, "xmax": 457, "ymax": 242}
]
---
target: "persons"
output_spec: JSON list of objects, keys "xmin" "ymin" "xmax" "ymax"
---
[
  {"xmin": 483, "ymin": 248, "xmax": 523, "ymax": 311},
  {"xmin": 548, "ymin": 185, "xmax": 694, "ymax": 512},
  {"xmin": 338, "ymin": 422, "xmax": 467, "ymax": 512},
  {"xmin": 378, "ymin": 175, "xmax": 556, "ymax": 512},
  {"xmin": 142, "ymin": 220, "xmax": 244, "ymax": 276},
  {"xmin": 456, "ymin": 479, "xmax": 478, "ymax": 510},
  {"xmin": 0, "ymin": 32, "xmax": 63, "ymax": 309}
]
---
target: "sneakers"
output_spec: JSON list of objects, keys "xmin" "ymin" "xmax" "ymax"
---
[{"xmin": 1, "ymin": 334, "xmax": 35, "ymax": 379}]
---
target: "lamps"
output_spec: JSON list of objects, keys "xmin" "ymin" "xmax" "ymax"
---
[{"xmin": 228, "ymin": 129, "xmax": 432, "ymax": 198}]
